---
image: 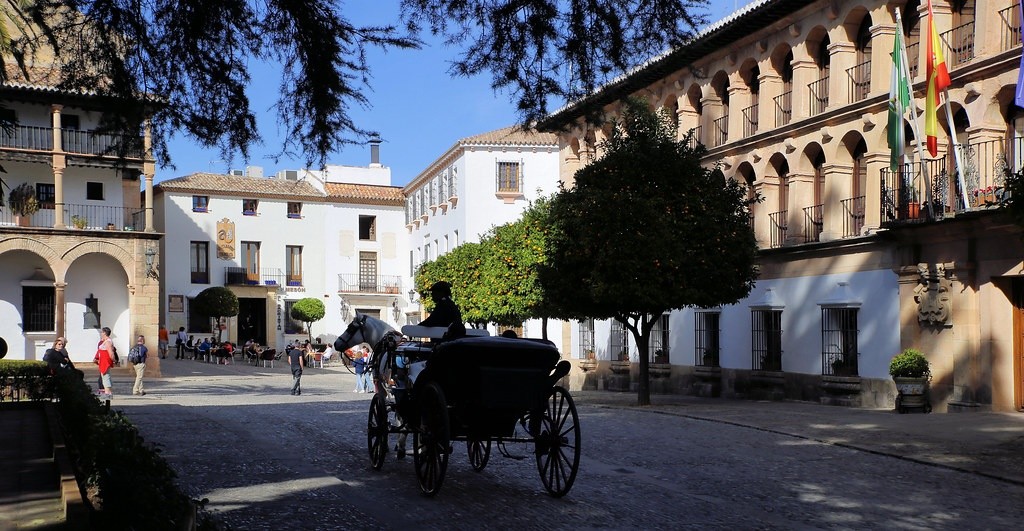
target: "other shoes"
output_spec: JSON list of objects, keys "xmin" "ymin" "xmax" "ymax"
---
[
  {"xmin": 99, "ymin": 394, "xmax": 113, "ymax": 400},
  {"xmin": 161, "ymin": 356, "xmax": 166, "ymax": 359},
  {"xmin": 140, "ymin": 392, "xmax": 146, "ymax": 395},
  {"xmin": 368, "ymin": 390, "xmax": 374, "ymax": 393}
]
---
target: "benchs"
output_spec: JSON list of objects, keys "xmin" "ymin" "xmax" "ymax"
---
[{"xmin": 401, "ymin": 325, "xmax": 489, "ymax": 364}]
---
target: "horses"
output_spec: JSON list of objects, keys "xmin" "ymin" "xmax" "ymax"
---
[{"xmin": 334, "ymin": 308, "xmax": 434, "ymax": 471}]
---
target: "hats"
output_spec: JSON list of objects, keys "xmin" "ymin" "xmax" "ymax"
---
[
  {"xmin": 59, "ymin": 337, "xmax": 68, "ymax": 342},
  {"xmin": 428, "ymin": 281, "xmax": 450, "ymax": 291}
]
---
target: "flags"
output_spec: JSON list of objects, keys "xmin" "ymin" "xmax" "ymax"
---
[
  {"xmin": 1013, "ymin": 0, "xmax": 1024, "ymax": 108},
  {"xmin": 924, "ymin": 0, "xmax": 951, "ymax": 158},
  {"xmin": 887, "ymin": 22, "xmax": 910, "ymax": 174}
]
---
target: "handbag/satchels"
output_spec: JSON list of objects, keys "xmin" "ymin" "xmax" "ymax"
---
[{"xmin": 176, "ymin": 332, "xmax": 182, "ymax": 344}]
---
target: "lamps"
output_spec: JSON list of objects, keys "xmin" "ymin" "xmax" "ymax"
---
[
  {"xmin": 407, "ymin": 290, "xmax": 418, "ymax": 304},
  {"xmin": 144, "ymin": 249, "xmax": 156, "ymax": 279}
]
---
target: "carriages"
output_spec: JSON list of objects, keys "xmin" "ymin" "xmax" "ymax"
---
[{"xmin": 333, "ymin": 308, "xmax": 582, "ymax": 496}]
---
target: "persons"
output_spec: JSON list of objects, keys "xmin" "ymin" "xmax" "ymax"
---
[
  {"xmin": 175, "ymin": 327, "xmax": 187, "ymax": 360},
  {"xmin": 42, "ymin": 337, "xmax": 75, "ymax": 373},
  {"xmin": 351, "ymin": 345, "xmax": 374, "ymax": 394},
  {"xmin": 159, "ymin": 324, "xmax": 169, "ymax": 359},
  {"xmin": 415, "ymin": 280, "xmax": 467, "ymax": 343},
  {"xmin": 128, "ymin": 334, "xmax": 148, "ymax": 395},
  {"xmin": 310, "ymin": 343, "xmax": 333, "ymax": 366},
  {"xmin": 245, "ymin": 339, "xmax": 254, "ymax": 363},
  {"xmin": 186, "ymin": 334, "xmax": 234, "ymax": 363},
  {"xmin": 93, "ymin": 326, "xmax": 118, "ymax": 400},
  {"xmin": 288, "ymin": 342, "xmax": 304, "ymax": 396},
  {"xmin": 292, "ymin": 339, "xmax": 313, "ymax": 351}
]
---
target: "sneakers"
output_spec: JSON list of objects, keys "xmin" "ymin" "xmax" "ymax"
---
[
  {"xmin": 359, "ymin": 390, "xmax": 365, "ymax": 393},
  {"xmin": 353, "ymin": 389, "xmax": 357, "ymax": 393}
]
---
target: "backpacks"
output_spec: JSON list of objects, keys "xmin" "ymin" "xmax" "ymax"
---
[{"xmin": 128, "ymin": 345, "xmax": 142, "ymax": 364}]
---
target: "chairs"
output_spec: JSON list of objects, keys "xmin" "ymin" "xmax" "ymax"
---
[{"xmin": 183, "ymin": 343, "xmax": 355, "ymax": 369}]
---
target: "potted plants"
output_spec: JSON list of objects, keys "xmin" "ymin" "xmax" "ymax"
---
[
  {"xmin": 655, "ymin": 349, "xmax": 665, "ymax": 363},
  {"xmin": 831, "ymin": 359, "xmax": 848, "ymax": 376},
  {"xmin": 617, "ymin": 352, "xmax": 630, "ymax": 360},
  {"xmin": 760, "ymin": 353, "xmax": 775, "ymax": 370},
  {"xmin": 107, "ymin": 222, "xmax": 115, "ymax": 230},
  {"xmin": 7, "ymin": 183, "xmax": 38, "ymax": 227},
  {"xmin": 960, "ymin": 184, "xmax": 1001, "ymax": 208},
  {"xmin": 903, "ymin": 187, "xmax": 920, "ymax": 219},
  {"xmin": 703, "ymin": 350, "xmax": 715, "ymax": 366},
  {"xmin": 888, "ymin": 348, "xmax": 931, "ymax": 396},
  {"xmin": 583, "ymin": 350, "xmax": 594, "ymax": 359},
  {"xmin": 71, "ymin": 215, "xmax": 87, "ymax": 229}
]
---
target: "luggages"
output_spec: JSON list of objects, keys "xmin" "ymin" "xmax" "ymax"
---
[{"xmin": 291, "ymin": 392, "xmax": 301, "ymax": 395}]
